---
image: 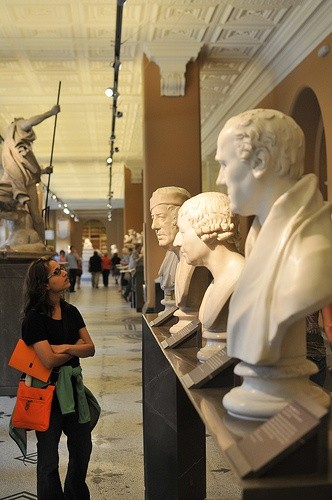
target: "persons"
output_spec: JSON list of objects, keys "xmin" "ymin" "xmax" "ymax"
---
[
  {"xmin": 120, "ymin": 243, "xmax": 143, "ymax": 302},
  {"xmin": 111, "ymin": 253, "xmax": 122, "ymax": 284},
  {"xmin": 2, "ymin": 105, "xmax": 61, "ymax": 242},
  {"xmin": 21, "ymin": 257, "xmax": 95, "ymax": 500},
  {"xmin": 88, "ymin": 251, "xmax": 102, "ymax": 288},
  {"xmin": 100, "ymin": 253, "xmax": 112, "ymax": 287},
  {"xmin": 150, "ymin": 186, "xmax": 193, "ymax": 296},
  {"xmin": 306, "ymin": 303, "xmax": 332, "ymax": 388},
  {"xmin": 214, "ymin": 109, "xmax": 332, "ymax": 365},
  {"xmin": 54, "ymin": 246, "xmax": 82, "ymax": 293},
  {"xmin": 173, "ymin": 192, "xmax": 245, "ymax": 331}
]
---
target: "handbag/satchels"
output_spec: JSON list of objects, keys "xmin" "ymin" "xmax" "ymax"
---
[{"xmin": 10, "ymin": 381, "xmax": 56, "ymax": 432}]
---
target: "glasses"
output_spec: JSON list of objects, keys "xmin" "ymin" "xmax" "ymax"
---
[{"xmin": 47, "ymin": 264, "xmax": 64, "ymax": 280}]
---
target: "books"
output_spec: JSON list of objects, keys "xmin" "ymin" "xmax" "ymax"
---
[{"xmin": 8, "ymin": 339, "xmax": 53, "ymax": 383}]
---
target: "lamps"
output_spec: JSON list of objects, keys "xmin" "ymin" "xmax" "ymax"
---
[
  {"xmin": 106, "ymin": 110, "xmax": 123, "ymax": 223},
  {"xmin": 105, "ymin": 87, "xmax": 114, "ymax": 99}
]
